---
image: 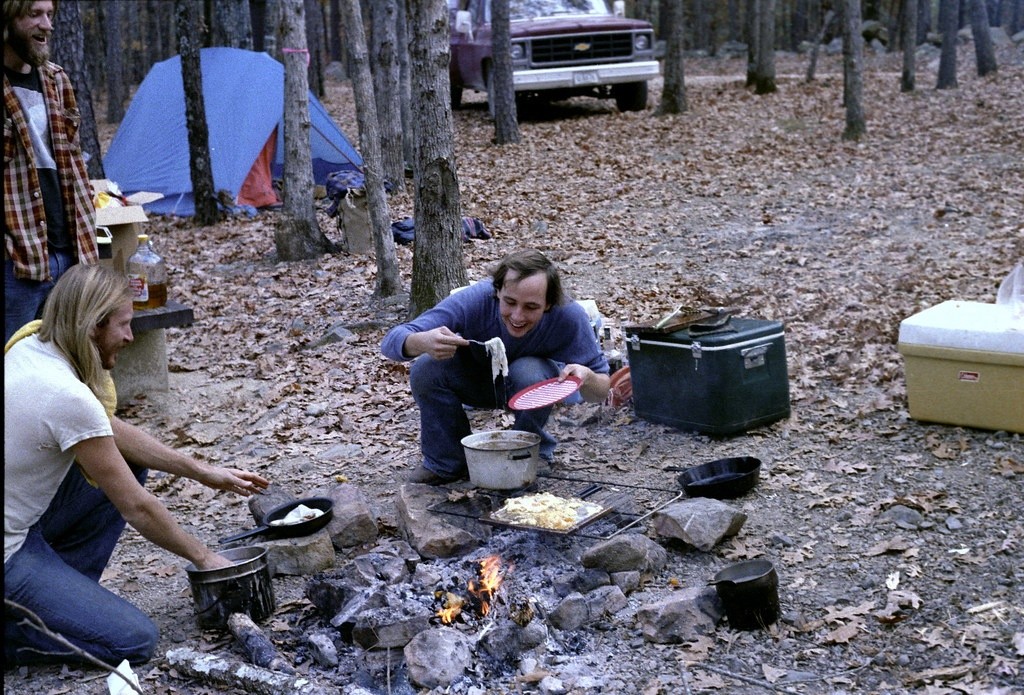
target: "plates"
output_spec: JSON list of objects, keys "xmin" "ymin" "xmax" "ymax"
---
[{"xmin": 508, "ymin": 376, "xmax": 583, "ymax": 411}]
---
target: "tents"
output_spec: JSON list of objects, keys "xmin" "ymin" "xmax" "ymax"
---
[{"xmin": 103, "ymin": 48, "xmax": 392, "ymax": 216}]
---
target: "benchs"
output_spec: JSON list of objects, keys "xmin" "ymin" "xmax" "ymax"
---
[{"xmin": 114, "ymin": 301, "xmax": 194, "ymax": 409}]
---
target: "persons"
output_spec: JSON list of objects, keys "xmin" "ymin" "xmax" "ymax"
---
[
  {"xmin": 4, "ymin": 266, "xmax": 270, "ymax": 670},
  {"xmin": 5, "ymin": 1, "xmax": 100, "ymax": 345},
  {"xmin": 381, "ymin": 250, "xmax": 611, "ymax": 485}
]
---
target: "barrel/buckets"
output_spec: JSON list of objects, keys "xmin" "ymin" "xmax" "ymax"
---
[{"xmin": 185, "ymin": 546, "xmax": 274, "ymax": 630}]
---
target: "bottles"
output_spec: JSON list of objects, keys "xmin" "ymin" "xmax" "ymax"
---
[{"xmin": 127, "ymin": 235, "xmax": 168, "ymax": 310}]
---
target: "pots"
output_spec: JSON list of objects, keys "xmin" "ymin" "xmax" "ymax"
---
[
  {"xmin": 218, "ymin": 498, "xmax": 334, "ymax": 546},
  {"xmin": 460, "ymin": 429, "xmax": 541, "ymax": 489},
  {"xmin": 663, "ymin": 455, "xmax": 762, "ymax": 501}
]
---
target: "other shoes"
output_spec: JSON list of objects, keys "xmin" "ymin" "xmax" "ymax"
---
[
  {"xmin": 540, "ymin": 453, "xmax": 556, "ymax": 469},
  {"xmin": 393, "ymin": 465, "xmax": 460, "ymax": 486}
]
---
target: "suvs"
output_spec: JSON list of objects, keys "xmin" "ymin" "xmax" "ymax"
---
[{"xmin": 448, "ymin": 1, "xmax": 661, "ymax": 116}]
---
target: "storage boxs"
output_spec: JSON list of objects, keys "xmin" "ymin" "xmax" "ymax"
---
[
  {"xmin": 89, "ymin": 178, "xmax": 164, "ymax": 270},
  {"xmin": 898, "ymin": 300, "xmax": 1024, "ymax": 436},
  {"xmin": 625, "ymin": 313, "xmax": 791, "ymax": 437}
]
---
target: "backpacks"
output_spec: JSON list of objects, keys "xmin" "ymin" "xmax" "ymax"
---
[{"xmin": 326, "ymin": 170, "xmax": 393, "ymax": 218}]
